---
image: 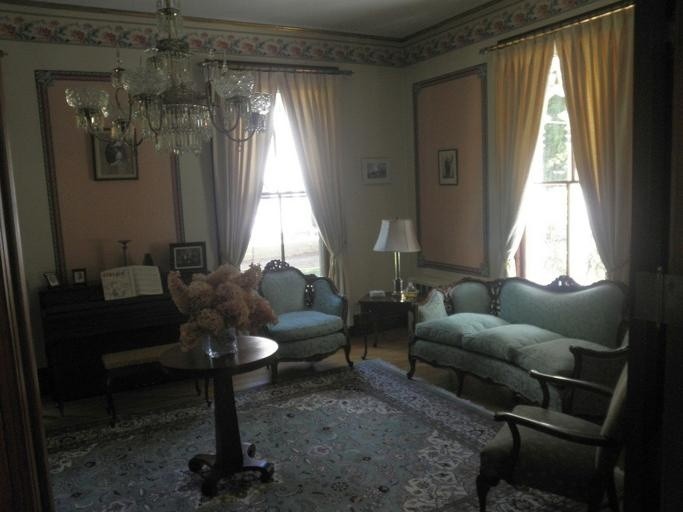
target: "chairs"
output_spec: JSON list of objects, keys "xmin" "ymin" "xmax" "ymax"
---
[
  {"xmin": 260, "ymin": 257, "xmax": 356, "ymax": 376},
  {"xmin": 473, "ymin": 363, "xmax": 631, "ymax": 511}
]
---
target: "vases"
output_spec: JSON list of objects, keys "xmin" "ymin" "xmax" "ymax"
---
[{"xmin": 199, "ymin": 327, "xmax": 239, "ymax": 357}]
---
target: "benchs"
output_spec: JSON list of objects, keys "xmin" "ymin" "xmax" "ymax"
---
[{"xmin": 100, "ymin": 345, "xmax": 213, "ymax": 427}]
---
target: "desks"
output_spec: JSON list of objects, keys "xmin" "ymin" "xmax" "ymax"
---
[
  {"xmin": 158, "ymin": 335, "xmax": 278, "ymax": 498},
  {"xmin": 358, "ymin": 293, "xmax": 413, "ymax": 362}
]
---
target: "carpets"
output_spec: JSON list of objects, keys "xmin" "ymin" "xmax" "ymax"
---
[{"xmin": 40, "ymin": 356, "xmax": 630, "ymax": 511}]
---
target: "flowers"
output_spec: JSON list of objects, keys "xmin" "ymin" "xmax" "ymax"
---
[{"xmin": 166, "ymin": 261, "xmax": 279, "ymax": 353}]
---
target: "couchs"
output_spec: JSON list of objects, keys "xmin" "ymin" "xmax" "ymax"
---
[{"xmin": 407, "ymin": 274, "xmax": 629, "ymax": 407}]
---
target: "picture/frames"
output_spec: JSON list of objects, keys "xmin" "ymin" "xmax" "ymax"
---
[
  {"xmin": 89, "ymin": 125, "xmax": 139, "ymax": 183},
  {"xmin": 436, "ymin": 146, "xmax": 459, "ymax": 187},
  {"xmin": 358, "ymin": 155, "xmax": 394, "ymax": 186},
  {"xmin": 42, "ymin": 271, "xmax": 60, "ymax": 290},
  {"xmin": 168, "ymin": 242, "xmax": 208, "ymax": 274},
  {"xmin": 71, "ymin": 266, "xmax": 88, "ymax": 289}
]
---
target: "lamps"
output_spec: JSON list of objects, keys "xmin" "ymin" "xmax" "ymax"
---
[
  {"xmin": 373, "ymin": 216, "xmax": 421, "ymax": 293},
  {"xmin": 62, "ymin": 1, "xmax": 270, "ymax": 166}
]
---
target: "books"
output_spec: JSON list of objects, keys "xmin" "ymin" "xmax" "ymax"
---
[{"xmin": 99, "ymin": 265, "xmax": 164, "ymax": 302}]
